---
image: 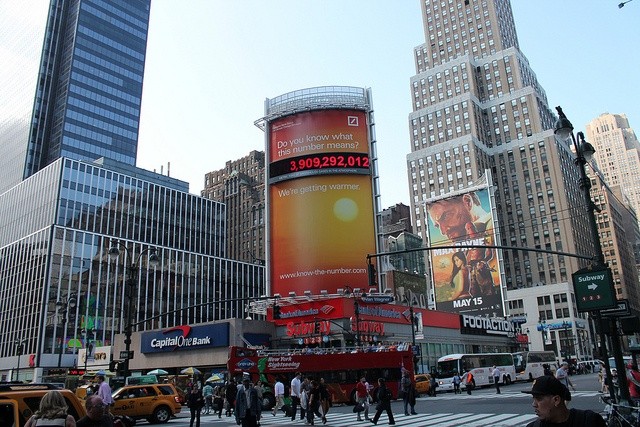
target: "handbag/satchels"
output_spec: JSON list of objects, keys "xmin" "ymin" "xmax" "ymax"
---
[{"xmin": 353, "ymin": 401, "xmax": 364, "ymax": 413}]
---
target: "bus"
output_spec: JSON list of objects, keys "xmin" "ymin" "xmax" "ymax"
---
[
  {"xmin": 512, "ymin": 351, "xmax": 558, "ymax": 382},
  {"xmin": 228, "ymin": 342, "xmax": 417, "ymax": 405},
  {"xmin": 435, "ymin": 352, "xmax": 516, "ymax": 392}
]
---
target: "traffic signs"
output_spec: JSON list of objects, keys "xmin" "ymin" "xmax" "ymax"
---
[{"xmin": 571, "ymin": 267, "xmax": 620, "ymax": 314}]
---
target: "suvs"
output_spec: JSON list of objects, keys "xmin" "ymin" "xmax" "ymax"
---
[
  {"xmin": 1, "ymin": 382, "xmax": 87, "ymax": 427},
  {"xmin": 82, "ymin": 383, "xmax": 182, "ymax": 424},
  {"xmin": 414, "ymin": 374, "xmax": 432, "ymax": 399}
]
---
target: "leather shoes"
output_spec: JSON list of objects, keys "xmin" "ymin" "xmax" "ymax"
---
[
  {"xmin": 412, "ymin": 412, "xmax": 418, "ymax": 415},
  {"xmin": 388, "ymin": 422, "xmax": 395, "ymax": 424},
  {"xmin": 370, "ymin": 419, "xmax": 377, "ymax": 424},
  {"xmin": 405, "ymin": 412, "xmax": 409, "ymax": 416}
]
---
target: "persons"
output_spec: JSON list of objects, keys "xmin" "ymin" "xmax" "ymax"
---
[
  {"xmin": 461, "ymin": 370, "xmax": 473, "ymax": 395},
  {"xmin": 492, "ymin": 365, "xmax": 501, "ymax": 394},
  {"xmin": 362, "ymin": 376, "xmax": 373, "ymax": 412},
  {"xmin": 462, "ymin": 223, "xmax": 493, "ymax": 296},
  {"xmin": 307, "ymin": 376, "xmax": 314, "ymax": 391},
  {"xmin": 316, "ymin": 378, "xmax": 334, "ymax": 423},
  {"xmin": 429, "ymin": 374, "xmax": 436, "ymax": 396},
  {"xmin": 547, "ymin": 364, "xmax": 553, "ymax": 374},
  {"xmin": 355, "ymin": 376, "xmax": 373, "ymax": 421},
  {"xmin": 22, "ymin": 391, "xmax": 76, "ymax": 427},
  {"xmin": 598, "ymin": 363, "xmax": 607, "ymax": 391},
  {"xmin": 213, "ymin": 384, "xmax": 224, "ymax": 418},
  {"xmin": 87, "ymin": 384, "xmax": 97, "ymax": 395},
  {"xmin": 300, "ymin": 377, "xmax": 310, "ymax": 420},
  {"xmin": 555, "ymin": 362, "xmax": 569, "ymax": 388},
  {"xmin": 451, "ymin": 373, "xmax": 461, "ymax": 394},
  {"xmin": 254, "ymin": 380, "xmax": 263, "ymax": 418},
  {"xmin": 522, "ymin": 376, "xmax": 607, "ymax": 427},
  {"xmin": 599, "ymin": 364, "xmax": 610, "ymax": 394},
  {"xmin": 401, "ymin": 369, "xmax": 418, "ymax": 415},
  {"xmin": 544, "ymin": 364, "xmax": 550, "ymax": 375},
  {"xmin": 370, "ymin": 378, "xmax": 396, "ymax": 424},
  {"xmin": 448, "ymin": 253, "xmax": 471, "ymax": 300},
  {"xmin": 202, "ymin": 375, "xmax": 207, "ymax": 385},
  {"xmin": 626, "ymin": 360, "xmax": 640, "ymax": 398},
  {"xmin": 290, "ymin": 371, "xmax": 306, "ymax": 421},
  {"xmin": 95, "ymin": 374, "xmax": 114, "ymax": 419},
  {"xmin": 186, "ymin": 383, "xmax": 203, "ymax": 427},
  {"xmin": 225, "ymin": 380, "xmax": 237, "ymax": 416},
  {"xmin": 567, "ymin": 362, "xmax": 595, "ymax": 375},
  {"xmin": 202, "ymin": 382, "xmax": 213, "ymax": 415},
  {"xmin": 286, "ymin": 342, "xmax": 412, "ymax": 355},
  {"xmin": 111, "ymin": 385, "xmax": 115, "ymax": 393},
  {"xmin": 76, "ymin": 395, "xmax": 135, "ymax": 427},
  {"xmin": 425, "ymin": 193, "xmax": 498, "ymax": 253},
  {"xmin": 307, "ymin": 379, "xmax": 326, "ymax": 425},
  {"xmin": 214, "ymin": 384, "xmax": 220, "ymax": 393},
  {"xmin": 235, "ymin": 378, "xmax": 261, "ymax": 427},
  {"xmin": 272, "ymin": 379, "xmax": 287, "ymax": 416}
]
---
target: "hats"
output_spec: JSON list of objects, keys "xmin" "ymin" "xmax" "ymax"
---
[{"xmin": 522, "ymin": 376, "xmax": 565, "ymax": 396}]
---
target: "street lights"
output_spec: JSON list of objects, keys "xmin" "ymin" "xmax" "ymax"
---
[
  {"xmin": 13, "ymin": 339, "xmax": 29, "ymax": 382},
  {"xmin": 108, "ymin": 240, "xmax": 160, "ymax": 376},
  {"xmin": 404, "ymin": 308, "xmax": 418, "ymax": 375},
  {"xmin": 56, "ymin": 295, "xmax": 78, "ymax": 367},
  {"xmin": 79, "ymin": 327, "xmax": 99, "ymax": 371},
  {"xmin": 343, "ymin": 286, "xmax": 368, "ymax": 347},
  {"xmin": 555, "ymin": 104, "xmax": 630, "ymax": 398}
]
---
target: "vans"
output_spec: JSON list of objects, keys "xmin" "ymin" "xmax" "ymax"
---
[
  {"xmin": 608, "ymin": 356, "xmax": 633, "ymax": 376},
  {"xmin": 586, "ymin": 360, "xmax": 604, "ymax": 370}
]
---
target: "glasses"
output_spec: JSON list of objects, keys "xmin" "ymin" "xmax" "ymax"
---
[{"xmin": 88, "ymin": 404, "xmax": 105, "ymax": 407}]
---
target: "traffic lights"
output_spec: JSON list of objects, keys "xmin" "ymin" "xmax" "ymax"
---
[
  {"xmin": 272, "ymin": 302, "xmax": 282, "ymax": 319},
  {"xmin": 368, "ymin": 264, "xmax": 377, "ymax": 285}
]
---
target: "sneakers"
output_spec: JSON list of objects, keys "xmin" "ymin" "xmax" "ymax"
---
[
  {"xmin": 358, "ymin": 419, "xmax": 363, "ymax": 421},
  {"xmin": 365, "ymin": 418, "xmax": 371, "ymax": 420}
]
